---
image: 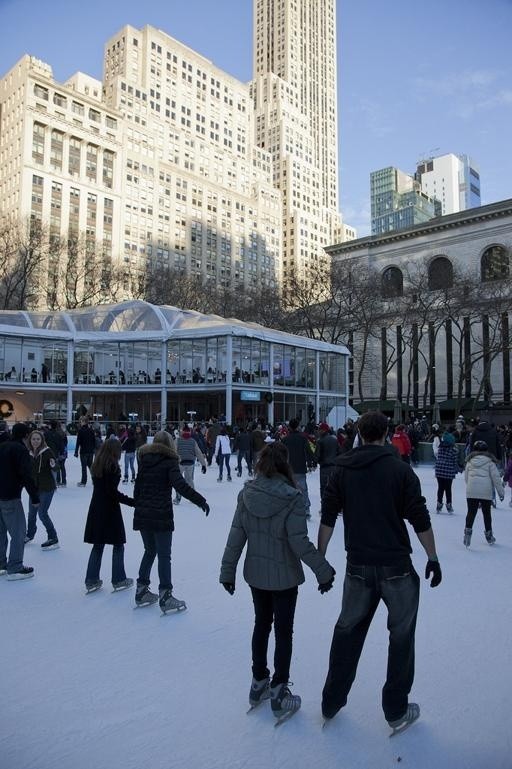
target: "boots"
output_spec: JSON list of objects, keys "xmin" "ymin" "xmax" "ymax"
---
[
  {"xmin": 86, "ymin": 577, "xmax": 185, "ymax": 610},
  {"xmin": 484, "ymin": 530, "xmax": 495, "ymax": 542},
  {"xmin": 249, "ymin": 678, "xmax": 301, "ymax": 717},
  {"xmin": 464, "ymin": 528, "xmax": 472, "ymax": 545}
]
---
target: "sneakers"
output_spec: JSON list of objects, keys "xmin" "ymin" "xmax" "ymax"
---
[
  {"xmin": 8, "ymin": 567, "xmax": 33, "ymax": 574},
  {"xmin": 41, "ymin": 537, "xmax": 57, "ymax": 547},
  {"xmin": 388, "ymin": 703, "xmax": 420, "ymax": 727},
  {"xmin": 24, "ymin": 535, "xmax": 33, "ymax": 543}
]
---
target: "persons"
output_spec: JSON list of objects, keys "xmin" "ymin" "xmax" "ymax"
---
[
  {"xmin": 219, "ymin": 443, "xmax": 336, "ymax": 729},
  {"xmin": 83, "ymin": 438, "xmax": 134, "ymax": 595},
  {"xmin": 318, "ymin": 410, "xmax": 442, "ymax": 738},
  {"xmin": 0, "ymin": 398, "xmax": 512, "ymax": 550},
  {"xmin": 0, "ymin": 422, "xmax": 43, "ymax": 581},
  {"xmin": 3, "ymin": 361, "xmax": 314, "ymax": 388},
  {"xmin": 133, "ymin": 430, "xmax": 210, "ymax": 618}
]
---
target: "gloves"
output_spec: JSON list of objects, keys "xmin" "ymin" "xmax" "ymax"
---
[
  {"xmin": 223, "ymin": 582, "xmax": 235, "ymax": 595},
  {"xmin": 318, "ymin": 572, "xmax": 335, "ymax": 594},
  {"xmin": 201, "ymin": 503, "xmax": 210, "ymax": 516},
  {"xmin": 425, "ymin": 560, "xmax": 441, "ymax": 587}
]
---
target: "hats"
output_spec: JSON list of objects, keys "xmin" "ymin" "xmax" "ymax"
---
[{"xmin": 443, "ymin": 431, "xmax": 455, "ymax": 441}]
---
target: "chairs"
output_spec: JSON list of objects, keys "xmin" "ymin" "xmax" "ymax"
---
[{"xmin": 0, "ymin": 364, "xmax": 244, "ymax": 388}]
